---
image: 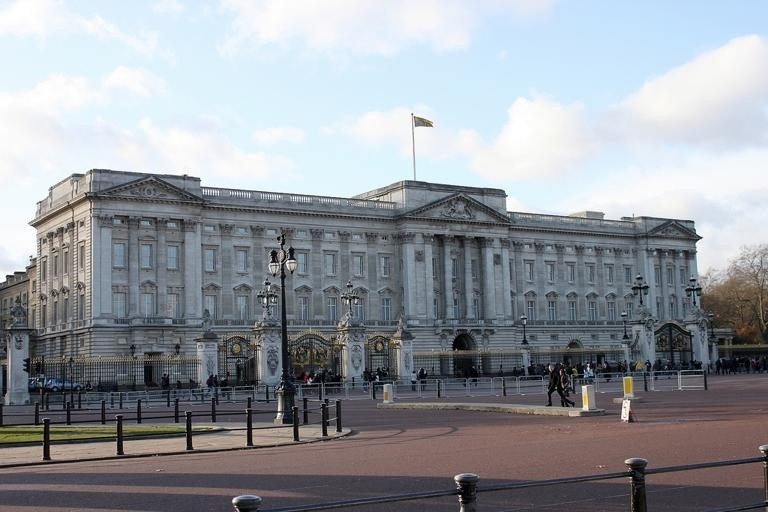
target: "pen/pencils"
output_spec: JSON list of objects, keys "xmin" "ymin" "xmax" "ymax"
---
[
  {"xmin": 581, "ymin": 385, "xmax": 597, "ymax": 409},
  {"xmin": 382, "ymin": 384, "xmax": 394, "ymax": 404}
]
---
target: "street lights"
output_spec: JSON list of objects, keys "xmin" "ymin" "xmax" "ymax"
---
[
  {"xmin": 129, "ymin": 344, "xmax": 136, "ymax": 391},
  {"xmin": 519, "ymin": 313, "xmax": 530, "ymax": 345},
  {"xmin": 266, "ymin": 228, "xmax": 297, "ymax": 424},
  {"xmin": 684, "ymin": 274, "xmax": 702, "ymax": 306},
  {"xmin": 708, "ymin": 310, "xmax": 718, "ymax": 338},
  {"xmin": 619, "ymin": 309, "xmax": 631, "ymax": 340},
  {"xmin": 257, "ymin": 276, "xmax": 279, "ymax": 311},
  {"xmin": 341, "ymin": 280, "xmax": 359, "ymax": 311},
  {"xmin": 632, "ymin": 273, "xmax": 649, "ymax": 305}
]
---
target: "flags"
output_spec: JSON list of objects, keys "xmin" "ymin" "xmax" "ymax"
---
[{"xmin": 414, "ymin": 116, "xmax": 434, "ymax": 128}]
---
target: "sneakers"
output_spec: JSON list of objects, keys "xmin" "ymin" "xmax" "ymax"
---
[{"xmin": 546, "ymin": 402, "xmax": 575, "ymax": 407}]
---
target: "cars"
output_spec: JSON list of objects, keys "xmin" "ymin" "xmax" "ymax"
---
[{"xmin": 29, "ymin": 377, "xmax": 94, "ymax": 392}]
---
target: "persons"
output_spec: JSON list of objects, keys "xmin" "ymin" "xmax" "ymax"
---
[
  {"xmin": 204, "ymin": 374, "xmax": 229, "ymax": 398},
  {"xmin": 544, "ymin": 364, "xmax": 566, "ymax": 407},
  {"xmin": 303, "ymin": 366, "xmax": 340, "ymax": 394},
  {"xmin": 418, "ymin": 367, "xmax": 427, "ymax": 391},
  {"xmin": 84, "ymin": 380, "xmax": 93, "ymax": 400},
  {"xmin": 497, "ymin": 364, "xmax": 504, "ymax": 377},
  {"xmin": 161, "ymin": 373, "xmax": 168, "ymax": 389},
  {"xmin": 513, "ymin": 356, "xmax": 704, "ymax": 384},
  {"xmin": 556, "ymin": 368, "xmax": 576, "ymax": 408},
  {"xmin": 714, "ymin": 354, "xmax": 768, "ymax": 376},
  {"xmin": 362, "ymin": 366, "xmax": 389, "ymax": 393},
  {"xmin": 411, "ymin": 370, "xmax": 418, "ymax": 391}
]
